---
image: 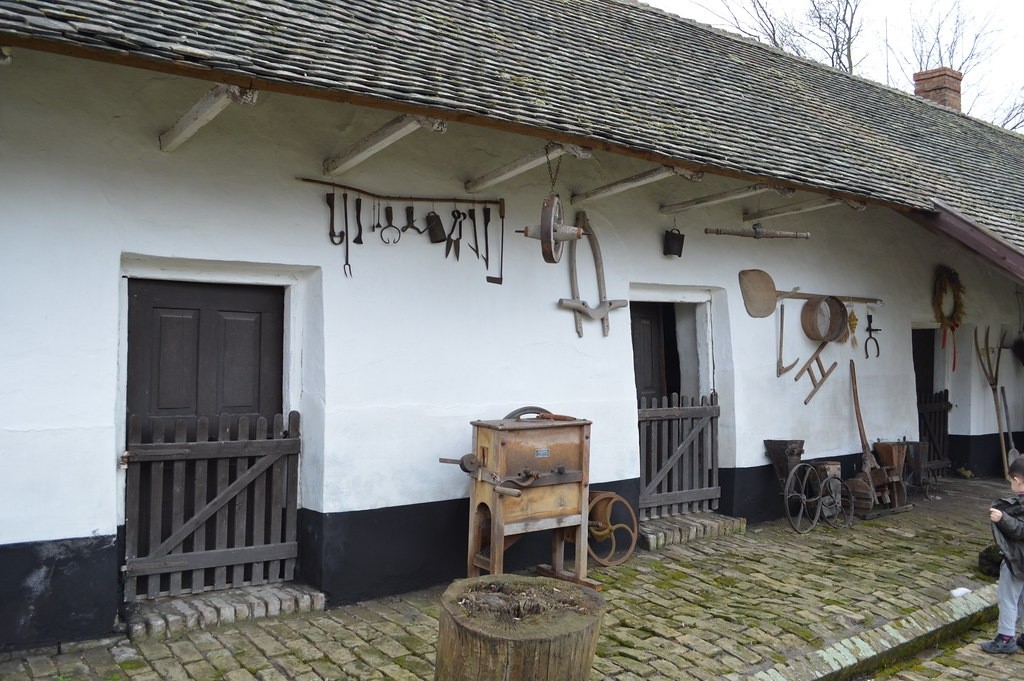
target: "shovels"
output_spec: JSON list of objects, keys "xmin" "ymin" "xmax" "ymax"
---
[{"xmin": 740, "ymin": 270, "xmax": 884, "ymax": 317}]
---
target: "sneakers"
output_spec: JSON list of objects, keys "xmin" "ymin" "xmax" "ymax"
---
[
  {"xmin": 1016, "ymin": 633, "xmax": 1024, "ymax": 647},
  {"xmin": 981, "ymin": 634, "xmax": 1017, "ymax": 653}
]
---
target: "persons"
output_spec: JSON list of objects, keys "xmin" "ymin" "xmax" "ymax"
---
[{"xmin": 981, "ymin": 454, "xmax": 1024, "ymax": 654}]
